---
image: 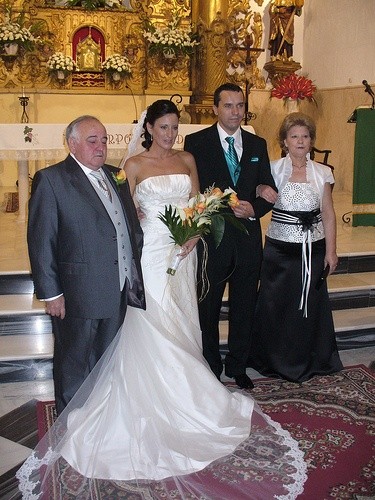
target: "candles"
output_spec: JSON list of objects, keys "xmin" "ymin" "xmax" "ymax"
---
[{"xmin": 22, "ymin": 85, "xmax": 25, "ymax": 97}]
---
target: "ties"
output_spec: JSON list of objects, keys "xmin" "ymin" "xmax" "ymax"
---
[
  {"xmin": 90, "ymin": 171, "xmax": 112, "ymax": 203},
  {"xmin": 225, "ymin": 137, "xmax": 240, "ymax": 185}
]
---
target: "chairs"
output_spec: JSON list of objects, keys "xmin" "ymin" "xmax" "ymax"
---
[{"xmin": 280, "ymin": 145, "xmax": 335, "ymax": 193}]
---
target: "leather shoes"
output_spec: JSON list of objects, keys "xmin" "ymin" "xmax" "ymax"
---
[{"xmin": 224, "ymin": 365, "xmax": 254, "ymax": 389}]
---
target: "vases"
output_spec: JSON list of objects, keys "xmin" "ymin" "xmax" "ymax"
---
[
  {"xmin": 287, "ymin": 98, "xmax": 298, "ymax": 115},
  {"xmin": 58, "ymin": 72, "xmax": 64, "ymax": 80},
  {"xmin": 3, "ymin": 43, "xmax": 18, "ymax": 55},
  {"xmin": 164, "ymin": 48, "xmax": 174, "ymax": 57},
  {"xmin": 113, "ymin": 73, "xmax": 120, "ymax": 82}
]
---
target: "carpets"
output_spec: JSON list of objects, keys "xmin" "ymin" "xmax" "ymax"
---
[{"xmin": 37, "ymin": 363, "xmax": 375, "ymax": 500}]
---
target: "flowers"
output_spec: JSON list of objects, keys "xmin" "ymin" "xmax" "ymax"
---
[
  {"xmin": 99, "ymin": 54, "xmax": 132, "ymax": 75},
  {"xmin": 45, "ymin": 52, "xmax": 79, "ymax": 73},
  {"xmin": 0, "ymin": 7, "xmax": 53, "ymax": 52},
  {"xmin": 157, "ymin": 182, "xmax": 251, "ymax": 251},
  {"xmin": 111, "ymin": 170, "xmax": 126, "ymax": 185},
  {"xmin": 142, "ymin": 15, "xmax": 202, "ymax": 57},
  {"xmin": 269, "ymin": 73, "xmax": 318, "ymax": 107}
]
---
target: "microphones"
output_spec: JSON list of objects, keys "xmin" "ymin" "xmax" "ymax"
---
[
  {"xmin": 362, "ymin": 80, "xmax": 374, "ymax": 95},
  {"xmin": 125, "ymin": 85, "xmax": 138, "ymax": 124}
]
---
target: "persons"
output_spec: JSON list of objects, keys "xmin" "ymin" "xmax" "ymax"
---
[
  {"xmin": 256, "ymin": 111, "xmax": 344, "ymax": 384},
  {"xmin": 267, "ymin": 0, "xmax": 304, "ymax": 61},
  {"xmin": 26, "ymin": 115, "xmax": 147, "ymax": 420},
  {"xmin": 184, "ymin": 83, "xmax": 277, "ymax": 389},
  {"xmin": 15, "ymin": 99, "xmax": 308, "ymax": 500}
]
